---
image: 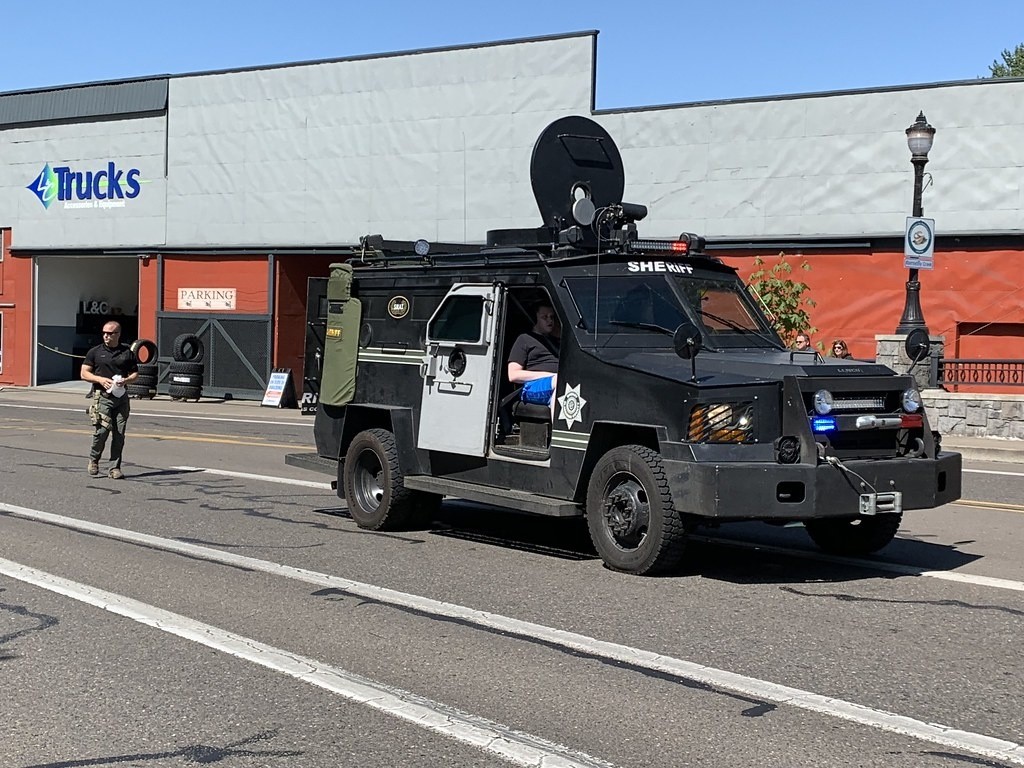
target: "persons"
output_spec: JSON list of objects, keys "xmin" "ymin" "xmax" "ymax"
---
[
  {"xmin": 795, "ymin": 334, "xmax": 814, "ymax": 351},
  {"xmin": 81, "ymin": 321, "xmax": 139, "ymax": 479},
  {"xmin": 507, "ymin": 300, "xmax": 560, "ymax": 424},
  {"xmin": 829, "ymin": 340, "xmax": 854, "ymax": 360}
]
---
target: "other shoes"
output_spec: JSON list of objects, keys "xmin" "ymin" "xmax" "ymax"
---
[
  {"xmin": 87, "ymin": 457, "xmax": 99, "ymax": 475},
  {"xmin": 108, "ymin": 468, "xmax": 125, "ymax": 479}
]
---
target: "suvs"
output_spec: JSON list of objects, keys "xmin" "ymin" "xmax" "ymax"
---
[{"xmin": 285, "ymin": 118, "xmax": 964, "ymax": 573}]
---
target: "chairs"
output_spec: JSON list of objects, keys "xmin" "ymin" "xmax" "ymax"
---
[{"xmin": 501, "ymin": 302, "xmax": 550, "ymax": 421}]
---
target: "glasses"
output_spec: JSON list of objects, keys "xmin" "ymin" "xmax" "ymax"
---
[{"xmin": 102, "ymin": 331, "xmax": 119, "ymax": 335}]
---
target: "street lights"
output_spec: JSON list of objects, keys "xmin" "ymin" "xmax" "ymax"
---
[{"xmin": 894, "ymin": 108, "xmax": 937, "ymax": 335}]
